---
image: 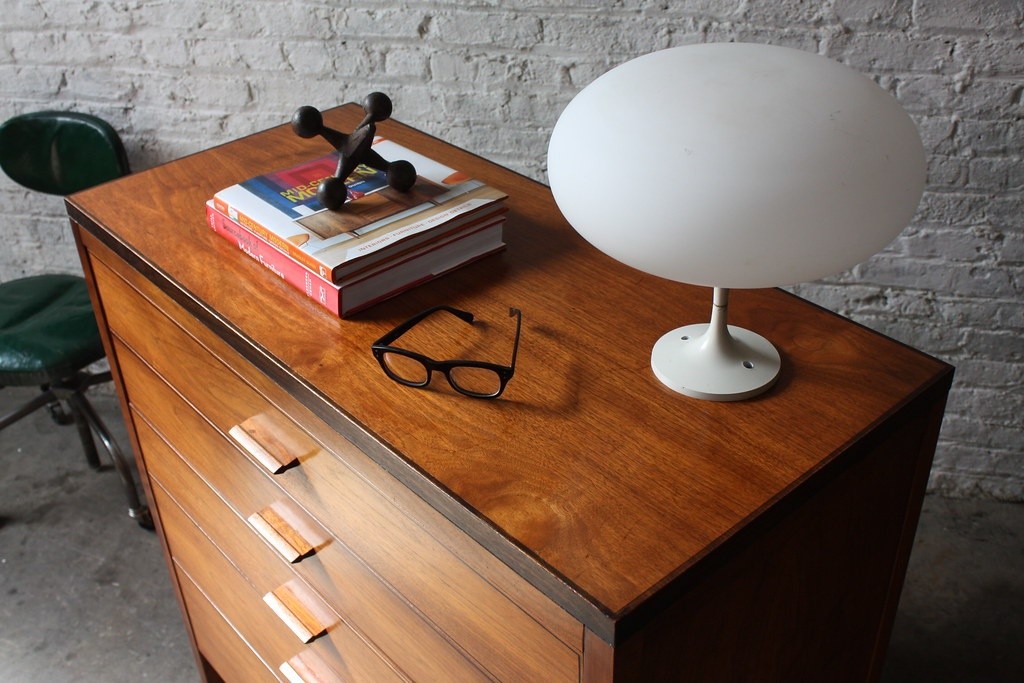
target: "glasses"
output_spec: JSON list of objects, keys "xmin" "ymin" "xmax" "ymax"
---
[{"xmin": 372, "ymin": 305, "xmax": 521, "ymax": 398}]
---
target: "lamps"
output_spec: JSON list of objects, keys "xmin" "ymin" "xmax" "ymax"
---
[{"xmin": 548, "ymin": 41, "xmax": 927, "ymax": 402}]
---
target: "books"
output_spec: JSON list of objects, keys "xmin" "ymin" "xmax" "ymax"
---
[{"xmin": 205, "ymin": 135, "xmax": 508, "ymax": 320}]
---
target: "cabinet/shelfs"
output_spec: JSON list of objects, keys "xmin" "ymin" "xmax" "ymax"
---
[{"xmin": 62, "ymin": 99, "xmax": 955, "ymax": 681}]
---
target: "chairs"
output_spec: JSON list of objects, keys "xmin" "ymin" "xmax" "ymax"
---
[{"xmin": 1, "ymin": 111, "xmax": 156, "ymax": 531}]
